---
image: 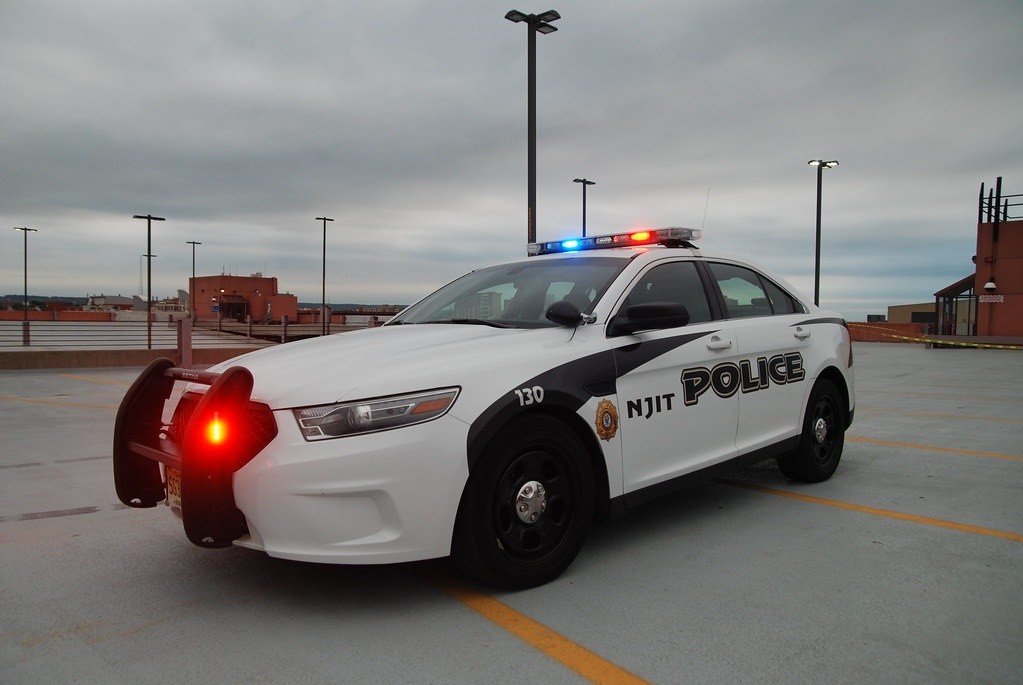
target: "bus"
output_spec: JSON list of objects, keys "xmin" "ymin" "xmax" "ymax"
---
[{"xmin": 154, "ymin": 301, "xmax": 182, "ymax": 311}]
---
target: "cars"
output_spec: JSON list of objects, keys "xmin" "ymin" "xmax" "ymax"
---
[{"xmin": 112, "ymin": 228, "xmax": 853, "ymax": 590}]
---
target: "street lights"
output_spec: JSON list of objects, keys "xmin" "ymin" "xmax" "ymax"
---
[
  {"xmin": 808, "ymin": 158, "xmax": 841, "ymax": 307},
  {"xmin": 315, "ymin": 216, "xmax": 335, "ymax": 336},
  {"xmin": 185, "ymin": 241, "xmax": 202, "ymax": 329},
  {"xmin": 133, "ymin": 214, "xmax": 167, "ymax": 349},
  {"xmin": 13, "ymin": 227, "xmax": 38, "ymax": 320},
  {"xmin": 572, "ymin": 176, "xmax": 597, "ymax": 237},
  {"xmin": 504, "ymin": 8, "xmax": 562, "ymax": 258}
]
